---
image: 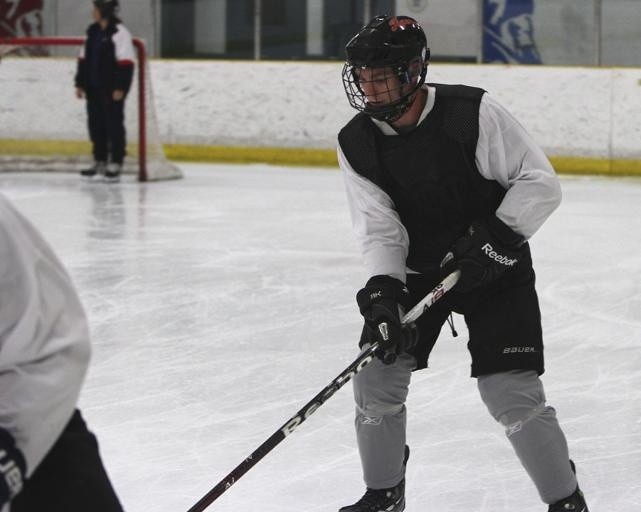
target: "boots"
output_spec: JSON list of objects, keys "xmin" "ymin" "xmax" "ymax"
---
[
  {"xmin": 547, "ymin": 460, "xmax": 588, "ymax": 512},
  {"xmin": 339, "ymin": 445, "xmax": 409, "ymax": 512}
]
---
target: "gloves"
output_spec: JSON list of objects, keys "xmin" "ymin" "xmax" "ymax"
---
[
  {"xmin": 356, "ymin": 274, "xmax": 418, "ymax": 366},
  {"xmin": 440, "ymin": 213, "xmax": 525, "ymax": 293}
]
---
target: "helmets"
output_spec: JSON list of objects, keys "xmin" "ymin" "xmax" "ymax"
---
[
  {"xmin": 339, "ymin": 470, "xmax": 345, "ymax": 486},
  {"xmin": 93, "ymin": 1, "xmax": 117, "ymax": 19},
  {"xmin": 345, "ymin": 13, "xmax": 428, "ymax": 66}
]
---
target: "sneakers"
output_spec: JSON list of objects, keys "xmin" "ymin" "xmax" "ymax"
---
[{"xmin": 80, "ymin": 161, "xmax": 121, "ymax": 177}]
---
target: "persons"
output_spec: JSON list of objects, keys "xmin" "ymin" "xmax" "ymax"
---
[
  {"xmin": 334, "ymin": 11, "xmax": 590, "ymax": 512},
  {"xmin": 0, "ymin": 192, "xmax": 125, "ymax": 512},
  {"xmin": 72, "ymin": 0, "xmax": 136, "ymax": 178}
]
